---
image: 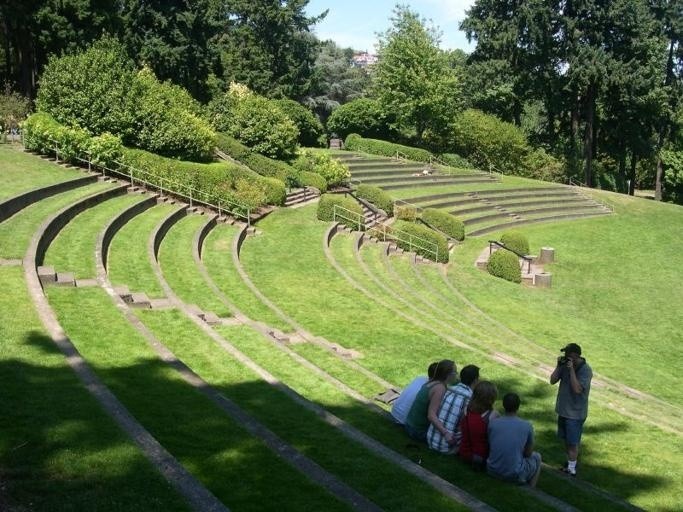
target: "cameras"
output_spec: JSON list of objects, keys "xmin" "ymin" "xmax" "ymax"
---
[{"xmin": 558, "ymin": 357, "xmax": 568, "ymax": 365}]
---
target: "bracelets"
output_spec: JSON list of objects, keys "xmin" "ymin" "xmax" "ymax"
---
[{"xmin": 441, "ymin": 428, "xmax": 448, "ymax": 435}]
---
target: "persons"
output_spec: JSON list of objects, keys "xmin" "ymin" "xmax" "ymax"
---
[
  {"xmin": 484, "ymin": 391, "xmax": 542, "ymax": 490},
  {"xmin": 388, "ymin": 361, "xmax": 438, "ymax": 429},
  {"xmin": 458, "ymin": 380, "xmax": 502, "ymax": 469},
  {"xmin": 550, "ymin": 343, "xmax": 593, "ymax": 477},
  {"xmin": 402, "ymin": 358, "xmax": 458, "ymax": 447},
  {"xmin": 425, "ymin": 363, "xmax": 480, "ymax": 456}
]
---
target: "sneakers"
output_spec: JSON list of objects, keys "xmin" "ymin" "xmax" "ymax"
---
[{"xmin": 557, "ymin": 462, "xmax": 577, "ymax": 480}]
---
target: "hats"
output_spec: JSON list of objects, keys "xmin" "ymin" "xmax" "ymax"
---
[{"xmin": 560, "ymin": 343, "xmax": 581, "ymax": 355}]
